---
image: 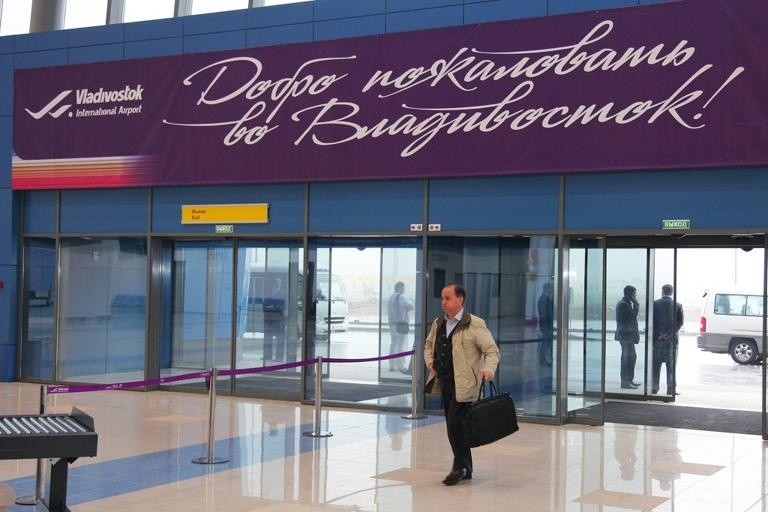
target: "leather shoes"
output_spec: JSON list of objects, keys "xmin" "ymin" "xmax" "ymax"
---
[
  {"xmin": 261, "ymin": 356, "xmax": 284, "ymax": 363},
  {"xmin": 443, "ymin": 467, "xmax": 472, "ymax": 485},
  {"xmin": 629, "ymin": 383, "xmax": 641, "ymax": 389},
  {"xmin": 675, "ymin": 392, "xmax": 680, "ymax": 395}
]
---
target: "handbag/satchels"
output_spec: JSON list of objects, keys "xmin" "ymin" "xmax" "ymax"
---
[
  {"xmin": 453, "ymin": 376, "xmax": 520, "ymax": 448},
  {"xmin": 397, "ymin": 322, "xmax": 409, "ymax": 333}
]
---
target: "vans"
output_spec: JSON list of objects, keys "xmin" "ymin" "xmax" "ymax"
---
[{"xmin": 697, "ymin": 288, "xmax": 765, "ymax": 365}]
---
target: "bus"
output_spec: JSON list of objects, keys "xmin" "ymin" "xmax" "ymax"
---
[{"xmin": 245, "ymin": 263, "xmax": 349, "ymax": 342}]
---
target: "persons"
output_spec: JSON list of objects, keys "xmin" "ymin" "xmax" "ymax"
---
[
  {"xmin": 536, "ymin": 282, "xmax": 554, "ymax": 369},
  {"xmin": 386, "ymin": 281, "xmax": 414, "ymax": 373},
  {"xmin": 651, "ymin": 283, "xmax": 685, "ymax": 395},
  {"xmin": 422, "ymin": 286, "xmax": 502, "ymax": 485},
  {"xmin": 260, "ymin": 276, "xmax": 286, "ymax": 362},
  {"xmin": 615, "ymin": 286, "xmax": 643, "ymax": 389}
]
